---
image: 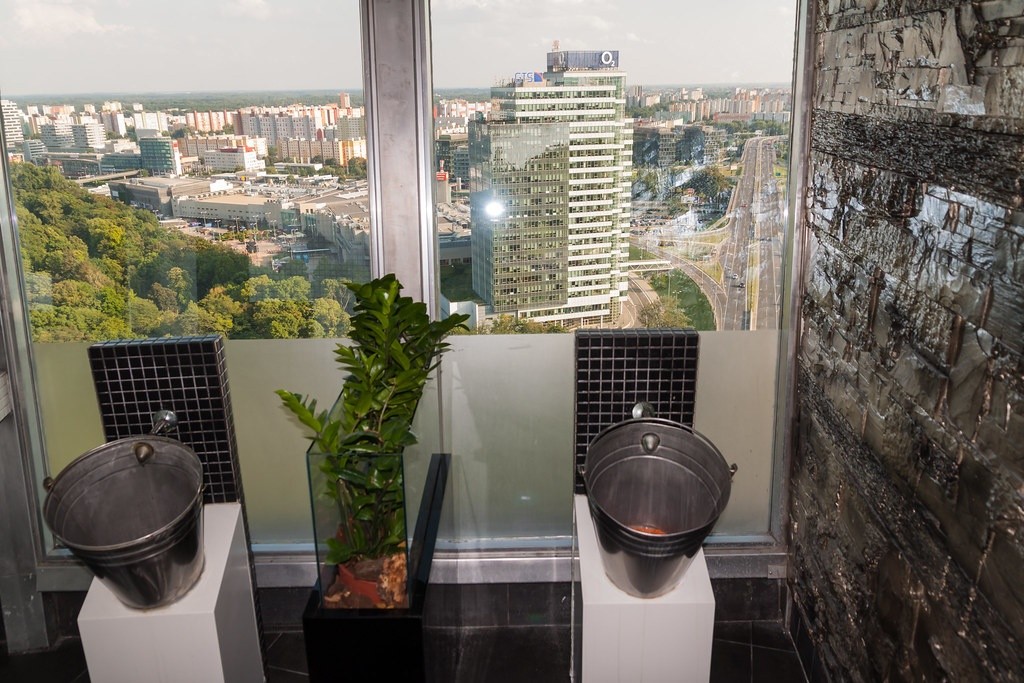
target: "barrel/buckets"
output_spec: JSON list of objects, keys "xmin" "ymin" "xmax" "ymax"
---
[
  {"xmin": 577, "ymin": 417, "xmax": 738, "ymax": 598},
  {"xmin": 41, "ymin": 434, "xmax": 207, "ymax": 609}
]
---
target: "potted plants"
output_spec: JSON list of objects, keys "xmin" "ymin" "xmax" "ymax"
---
[{"xmin": 273, "ymin": 274, "xmax": 473, "ymax": 603}]
